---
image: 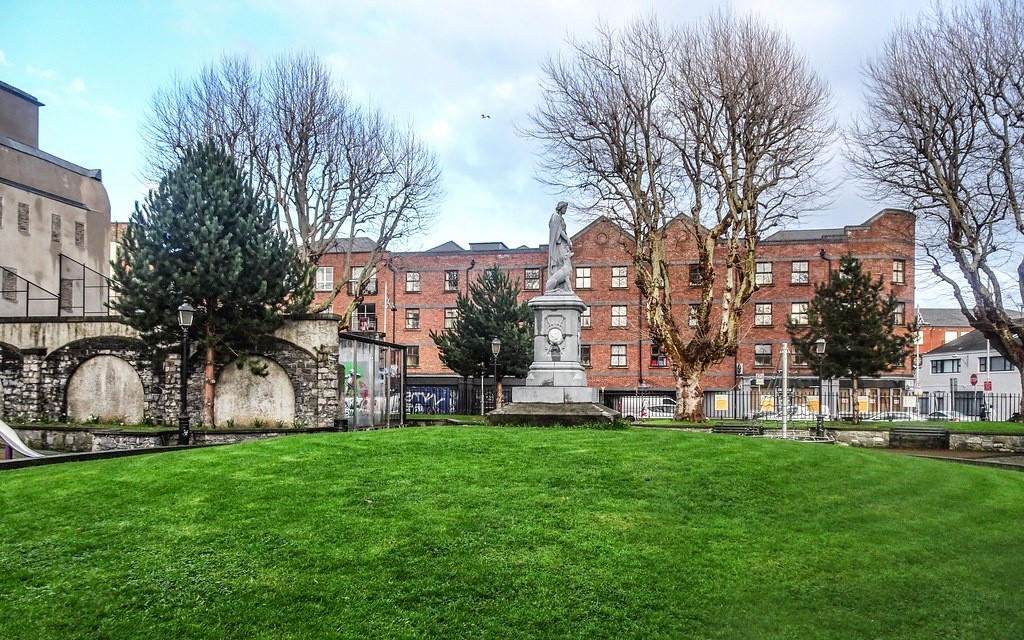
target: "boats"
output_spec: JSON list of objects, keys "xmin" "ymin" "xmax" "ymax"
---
[{"xmin": 739, "ymin": 342, "xmax": 836, "ymax": 444}]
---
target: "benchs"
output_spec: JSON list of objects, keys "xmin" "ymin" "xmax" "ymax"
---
[
  {"xmin": 712, "ymin": 421, "xmax": 763, "ymax": 437},
  {"xmin": 888, "ymin": 428, "xmax": 949, "ymax": 451}
]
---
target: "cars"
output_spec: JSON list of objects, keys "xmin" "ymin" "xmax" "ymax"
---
[
  {"xmin": 756, "ymin": 406, "xmax": 832, "ymax": 422},
  {"xmin": 860, "ymin": 411, "xmax": 922, "ymax": 421},
  {"xmin": 926, "ymin": 411, "xmax": 972, "ymax": 422},
  {"xmin": 642, "ymin": 405, "xmax": 711, "ymax": 422}
]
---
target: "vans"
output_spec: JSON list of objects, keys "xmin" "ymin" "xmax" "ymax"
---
[{"xmin": 619, "ymin": 396, "xmax": 677, "ymax": 421}]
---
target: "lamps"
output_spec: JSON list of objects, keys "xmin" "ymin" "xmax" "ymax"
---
[{"xmin": 736, "ymin": 363, "xmax": 744, "ymax": 375}]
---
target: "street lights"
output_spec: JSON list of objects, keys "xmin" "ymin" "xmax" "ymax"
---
[
  {"xmin": 916, "ymin": 312, "xmax": 931, "ymax": 421},
  {"xmin": 816, "ymin": 338, "xmax": 827, "ymax": 436},
  {"xmin": 177, "ymin": 300, "xmax": 194, "ymax": 445},
  {"xmin": 490, "ymin": 336, "xmax": 500, "ymax": 410},
  {"xmin": 383, "ymin": 300, "xmax": 397, "ymax": 342}
]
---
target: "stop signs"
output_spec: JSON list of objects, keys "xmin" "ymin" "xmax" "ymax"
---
[{"xmin": 969, "ymin": 373, "xmax": 978, "ymax": 385}]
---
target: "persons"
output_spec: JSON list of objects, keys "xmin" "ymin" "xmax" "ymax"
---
[
  {"xmin": 979, "ymin": 404, "xmax": 996, "ymax": 421},
  {"xmin": 543, "ymin": 200, "xmax": 577, "ymax": 296}
]
